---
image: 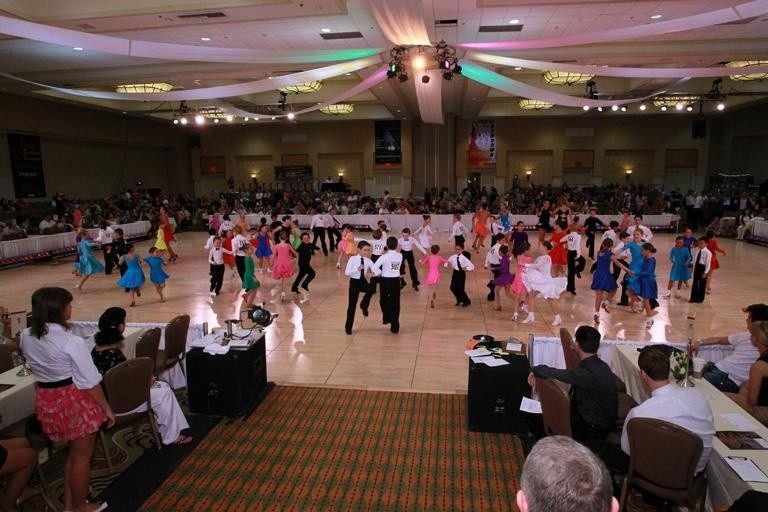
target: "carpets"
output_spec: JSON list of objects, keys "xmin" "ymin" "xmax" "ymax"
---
[{"xmin": 91, "ymin": 384, "xmax": 546, "ymax": 512}]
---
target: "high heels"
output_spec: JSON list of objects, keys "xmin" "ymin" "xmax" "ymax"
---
[
  {"xmin": 211, "ymin": 285, "xmax": 309, "ymax": 308},
  {"xmin": 487, "ymin": 283, "xmax": 711, "ymax": 330}
]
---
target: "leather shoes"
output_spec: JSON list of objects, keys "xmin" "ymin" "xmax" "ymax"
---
[
  {"xmin": 345, "ymin": 281, "xmax": 471, "ymax": 335},
  {"xmin": 95, "ymin": 497, "xmax": 111, "ymax": 512},
  {"xmin": 173, "ymin": 435, "xmax": 192, "ymax": 444}
]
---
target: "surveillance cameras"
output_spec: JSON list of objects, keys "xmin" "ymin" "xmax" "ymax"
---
[{"xmin": 135, "ymin": 174, "xmax": 145, "ymax": 186}]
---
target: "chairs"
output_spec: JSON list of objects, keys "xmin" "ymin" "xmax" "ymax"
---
[
  {"xmin": 534, "ymin": 327, "xmax": 708, "ymax": 512},
  {"xmin": 24, "ymin": 313, "xmax": 191, "ymax": 498}
]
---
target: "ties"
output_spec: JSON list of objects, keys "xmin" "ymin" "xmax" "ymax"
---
[
  {"xmin": 457, "ymin": 257, "xmax": 462, "ymax": 272},
  {"xmin": 360, "ymin": 258, "xmax": 364, "ymax": 284},
  {"xmin": 696, "ymin": 250, "xmax": 701, "ymax": 264}
]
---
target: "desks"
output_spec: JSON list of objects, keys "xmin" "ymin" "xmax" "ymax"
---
[
  {"xmin": 613, "ymin": 344, "xmax": 768, "ymax": 512},
  {"xmin": 0, "ymin": 327, "xmax": 143, "ymax": 430}
]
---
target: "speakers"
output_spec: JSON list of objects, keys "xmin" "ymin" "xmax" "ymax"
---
[
  {"xmin": 691, "ymin": 119, "xmax": 707, "ymax": 140},
  {"xmin": 190, "ymin": 134, "xmax": 203, "ymax": 151}
]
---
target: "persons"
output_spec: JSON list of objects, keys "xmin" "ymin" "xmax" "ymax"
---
[
  {"xmin": 0, "ymin": 333, "xmax": 25, "ymax": 374},
  {"xmin": 528, "ymin": 325, "xmax": 619, "ymax": 450},
  {"xmin": 690, "ymin": 303, "xmax": 767, "ymax": 393},
  {"xmin": 370, "ymin": 236, "xmax": 404, "ymax": 334},
  {"xmin": 91, "ymin": 307, "xmax": 194, "ymax": 447},
  {"xmin": 0, "ymin": 414, "xmax": 38, "ymax": 511},
  {"xmin": 1, "ymin": 172, "xmax": 766, "ymax": 324},
  {"xmin": 20, "ymin": 287, "xmax": 115, "ymax": 512},
  {"xmin": 343, "ymin": 241, "xmax": 381, "ymax": 336},
  {"xmin": 515, "ymin": 434, "xmax": 620, "ymax": 511},
  {"xmin": 723, "ymin": 320, "xmax": 768, "ymax": 425},
  {"xmin": 602, "ymin": 347, "xmax": 714, "ymax": 512}
]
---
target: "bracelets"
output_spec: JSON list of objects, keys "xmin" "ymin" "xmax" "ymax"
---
[{"xmin": 698, "ymin": 337, "xmax": 704, "ymax": 345}]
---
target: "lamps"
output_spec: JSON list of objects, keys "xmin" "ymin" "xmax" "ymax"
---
[
  {"xmin": 115, "ymin": 82, "xmax": 174, "ymax": 94},
  {"xmin": 383, "ymin": 46, "xmax": 411, "ymax": 83},
  {"xmin": 516, "ymin": 71, "xmax": 596, "ymax": 112},
  {"xmin": 653, "ymin": 94, "xmax": 699, "ymax": 108},
  {"xmin": 269, "ymin": 76, "xmax": 354, "ymax": 115},
  {"xmin": 724, "ymin": 61, "xmax": 768, "ymax": 82},
  {"xmin": 433, "ymin": 38, "xmax": 464, "ymax": 81}
]
